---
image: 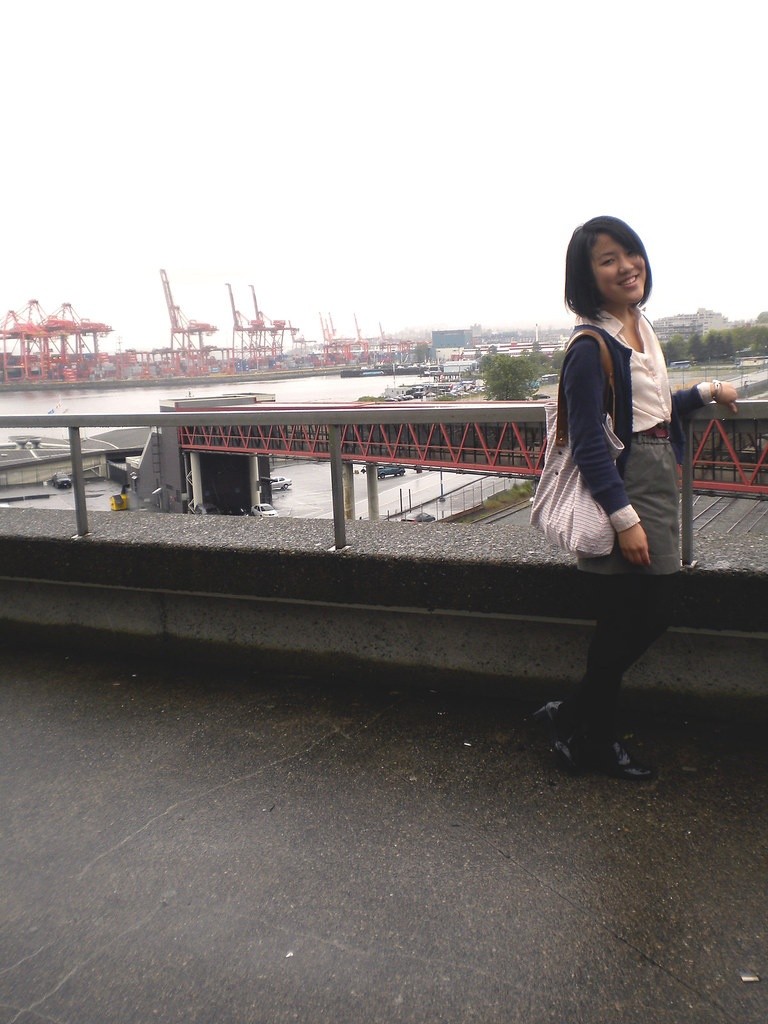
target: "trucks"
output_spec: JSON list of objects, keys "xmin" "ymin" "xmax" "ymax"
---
[{"xmin": 378, "ymin": 465, "xmax": 406, "ymax": 479}]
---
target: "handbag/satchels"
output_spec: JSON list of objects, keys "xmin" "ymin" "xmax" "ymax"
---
[{"xmin": 530, "ymin": 329, "xmax": 625, "ymax": 557}]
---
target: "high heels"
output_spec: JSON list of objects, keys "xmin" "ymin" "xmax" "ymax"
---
[
  {"xmin": 529, "ymin": 700, "xmax": 597, "ymax": 777},
  {"xmin": 594, "ymin": 741, "xmax": 652, "ymax": 779}
]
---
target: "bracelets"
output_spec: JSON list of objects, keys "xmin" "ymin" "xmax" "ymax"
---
[{"xmin": 712, "ymin": 380, "xmax": 722, "ymax": 401}]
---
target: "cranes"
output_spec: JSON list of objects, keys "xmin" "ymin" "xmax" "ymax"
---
[
  {"xmin": 225, "ymin": 281, "xmax": 431, "ymax": 374},
  {"xmin": 157, "ymin": 269, "xmax": 221, "ymax": 379},
  {"xmin": 0, "ymin": 298, "xmax": 116, "ymax": 385}
]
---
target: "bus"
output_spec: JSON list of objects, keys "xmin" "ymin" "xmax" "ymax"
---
[{"xmin": 669, "ymin": 360, "xmax": 691, "ymax": 370}]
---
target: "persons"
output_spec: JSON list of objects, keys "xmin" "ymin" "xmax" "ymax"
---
[{"xmin": 553, "ymin": 216, "xmax": 738, "ymax": 781}]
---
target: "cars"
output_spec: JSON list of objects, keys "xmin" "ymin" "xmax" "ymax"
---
[
  {"xmin": 270, "ymin": 476, "xmax": 293, "ymax": 491},
  {"xmin": 384, "ymin": 383, "xmax": 489, "ymax": 402},
  {"xmin": 251, "ymin": 503, "xmax": 279, "ymax": 517}
]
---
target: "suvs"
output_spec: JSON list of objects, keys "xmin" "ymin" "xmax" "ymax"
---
[{"xmin": 52, "ymin": 472, "xmax": 72, "ymax": 489}]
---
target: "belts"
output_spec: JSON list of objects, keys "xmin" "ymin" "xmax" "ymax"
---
[{"xmin": 638, "ymin": 425, "xmax": 670, "ymax": 439}]
